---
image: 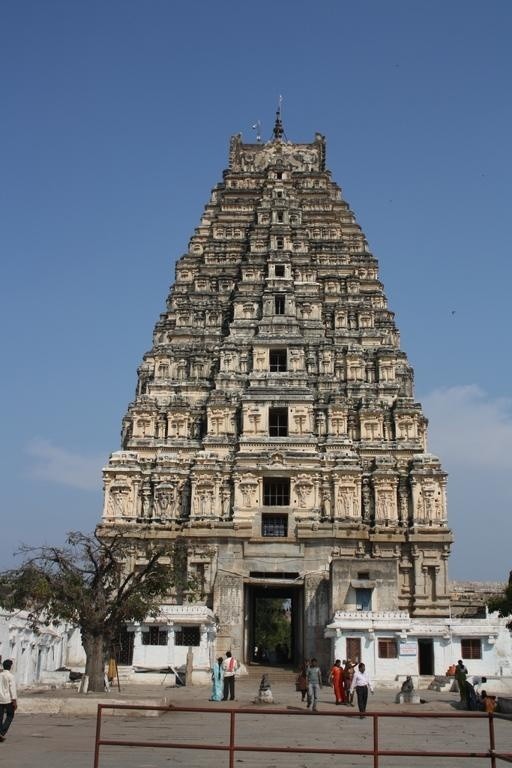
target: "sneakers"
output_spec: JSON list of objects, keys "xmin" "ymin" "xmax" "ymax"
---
[
  {"xmin": 306, "ymin": 704, "xmax": 319, "ymax": 714},
  {"xmin": 0, "ymin": 734, "xmax": 7, "ymax": 742},
  {"xmin": 208, "ymin": 697, "xmax": 235, "ymax": 701}
]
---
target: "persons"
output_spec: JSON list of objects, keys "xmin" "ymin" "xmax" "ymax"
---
[
  {"xmin": 454, "ymin": 659, "xmax": 469, "ymax": 689},
  {"xmin": 328, "ymin": 659, "xmax": 345, "ymax": 705},
  {"xmin": 208, "ymin": 656, "xmax": 225, "ymax": 701},
  {"xmin": 0, "ymin": 659, "xmax": 17, "ymax": 743},
  {"xmin": 222, "ymin": 650, "xmax": 240, "ymax": 701},
  {"xmin": 477, "ymin": 689, "xmax": 488, "ymax": 711},
  {"xmin": 305, "ymin": 658, "xmax": 323, "ymax": 713},
  {"xmin": 349, "ymin": 663, "xmax": 375, "ymax": 718},
  {"xmin": 297, "ymin": 658, "xmax": 310, "ymax": 702},
  {"xmin": 341, "ymin": 659, "xmax": 357, "ymax": 708}
]
